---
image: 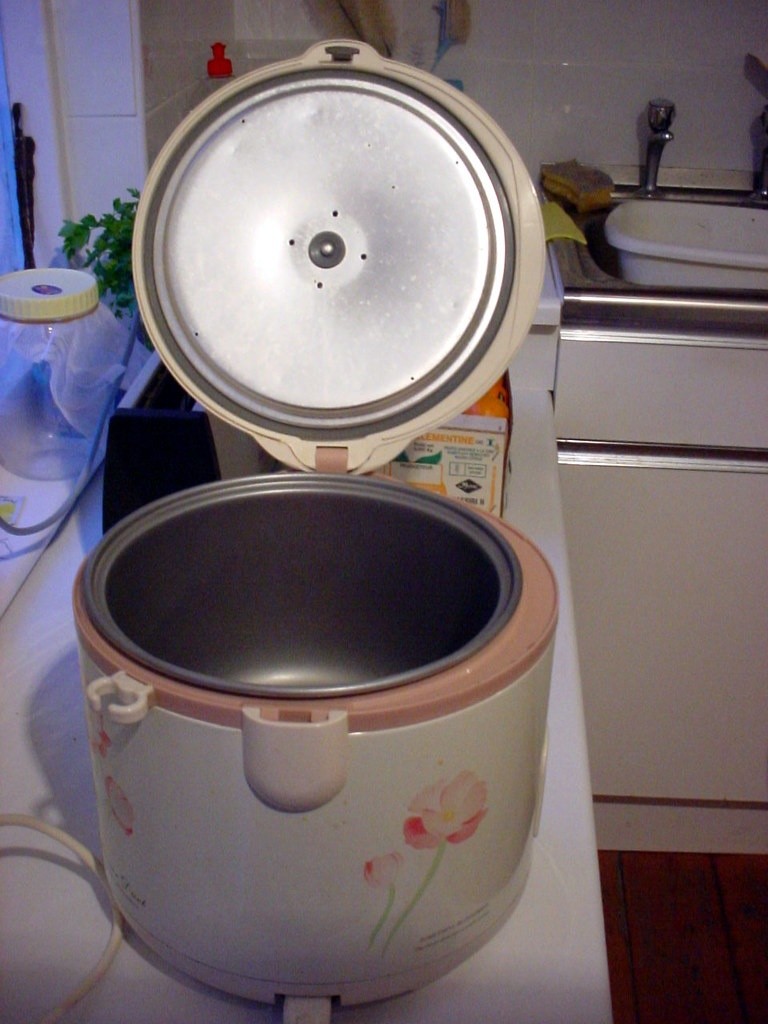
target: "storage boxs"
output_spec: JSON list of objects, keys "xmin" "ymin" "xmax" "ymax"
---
[
  {"xmin": 605, "ymin": 200, "xmax": 767, "ymax": 291},
  {"xmin": 192, "ymin": 368, "xmax": 514, "ymax": 517}
]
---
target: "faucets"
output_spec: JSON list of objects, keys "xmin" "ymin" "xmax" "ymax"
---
[{"xmin": 641, "ymin": 98, "xmax": 675, "ymax": 193}]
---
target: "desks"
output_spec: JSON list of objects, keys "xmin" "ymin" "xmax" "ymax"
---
[{"xmin": 0, "ymin": 390, "xmax": 616, "ymax": 1024}]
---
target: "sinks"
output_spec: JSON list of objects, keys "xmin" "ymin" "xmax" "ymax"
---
[{"xmin": 534, "ymin": 176, "xmax": 768, "ymax": 301}]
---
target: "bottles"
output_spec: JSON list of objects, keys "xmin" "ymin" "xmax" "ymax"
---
[
  {"xmin": 0, "ymin": 268, "xmax": 123, "ymax": 481},
  {"xmin": 184, "ymin": 43, "xmax": 237, "ymax": 109}
]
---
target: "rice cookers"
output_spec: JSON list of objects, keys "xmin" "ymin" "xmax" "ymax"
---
[{"xmin": 76, "ymin": 38, "xmax": 560, "ymax": 1004}]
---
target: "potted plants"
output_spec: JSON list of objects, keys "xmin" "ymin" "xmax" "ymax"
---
[{"xmin": 43, "ymin": 190, "xmax": 147, "ymax": 436}]
